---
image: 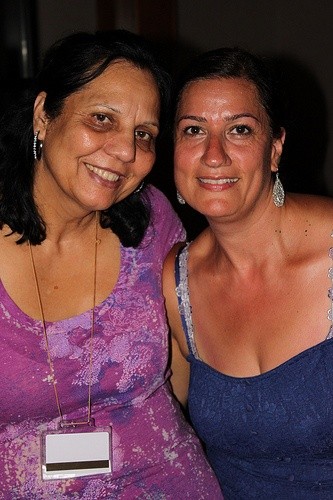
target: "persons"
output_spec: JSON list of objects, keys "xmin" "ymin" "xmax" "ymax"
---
[
  {"xmin": 164, "ymin": 46, "xmax": 333, "ymax": 500},
  {"xmin": 0, "ymin": 29, "xmax": 226, "ymax": 500}
]
